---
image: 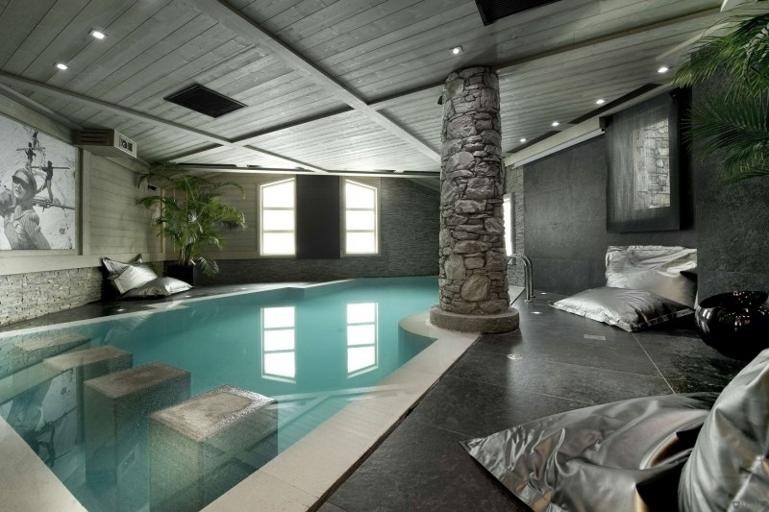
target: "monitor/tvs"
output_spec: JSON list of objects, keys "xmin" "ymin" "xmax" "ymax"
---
[{"xmin": 599, "ymin": 90, "xmax": 683, "ymax": 232}]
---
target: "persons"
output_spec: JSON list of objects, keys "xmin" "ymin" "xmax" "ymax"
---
[
  {"xmin": 4, "ymin": 376, "xmax": 59, "ymax": 471},
  {"xmin": 23, "ymin": 142, "xmax": 33, "ymax": 174},
  {"xmin": 33, "ymin": 160, "xmax": 53, "ymax": 206},
  {"xmin": 31, "ymin": 130, "xmax": 39, "ymax": 148},
  {"xmin": 0, "ymin": 168, "xmax": 52, "ymax": 249}
]
